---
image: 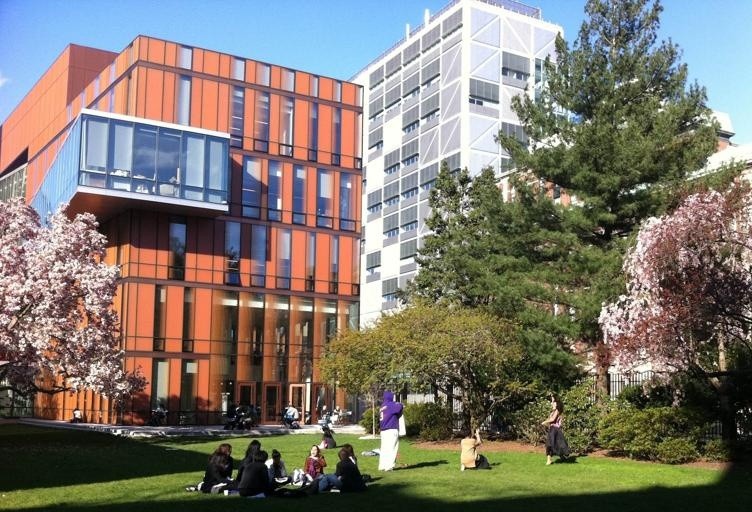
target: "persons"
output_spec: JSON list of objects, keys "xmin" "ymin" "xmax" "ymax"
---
[
  {"xmin": 73, "ymin": 408, "xmax": 82, "ymax": 423},
  {"xmin": 379, "ymin": 393, "xmax": 404, "ymax": 470},
  {"xmin": 460, "ymin": 429, "xmax": 490, "ymax": 471},
  {"xmin": 321, "ymin": 406, "xmax": 343, "ymax": 426},
  {"xmin": 542, "ymin": 395, "xmax": 570, "ymax": 464},
  {"xmin": 226, "ymin": 403, "xmax": 259, "ymax": 430},
  {"xmin": 319, "ymin": 427, "xmax": 336, "ymax": 449},
  {"xmin": 155, "ymin": 404, "xmax": 167, "ymax": 425},
  {"xmin": 198, "ymin": 440, "xmax": 368, "ymax": 498}
]
